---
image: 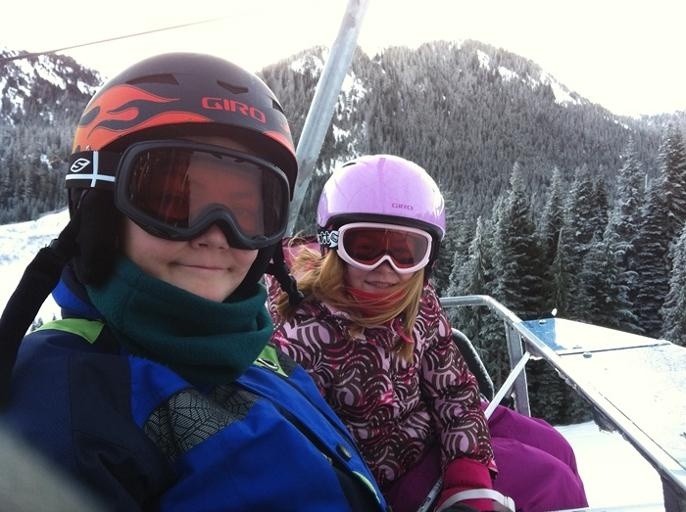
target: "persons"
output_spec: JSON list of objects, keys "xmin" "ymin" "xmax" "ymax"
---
[
  {"xmin": 0, "ymin": 47, "xmax": 394, "ymax": 511},
  {"xmin": 262, "ymin": 151, "xmax": 589, "ymax": 511}
]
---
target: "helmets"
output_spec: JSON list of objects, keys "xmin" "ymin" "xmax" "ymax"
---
[
  {"xmin": 315, "ymin": 151, "xmax": 445, "ymax": 241},
  {"xmin": 67, "ymin": 53, "xmax": 298, "ymax": 208}
]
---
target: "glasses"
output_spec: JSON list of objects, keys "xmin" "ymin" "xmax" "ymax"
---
[
  {"xmin": 317, "ymin": 223, "xmax": 436, "ymax": 273},
  {"xmin": 66, "ymin": 142, "xmax": 290, "ymax": 249}
]
